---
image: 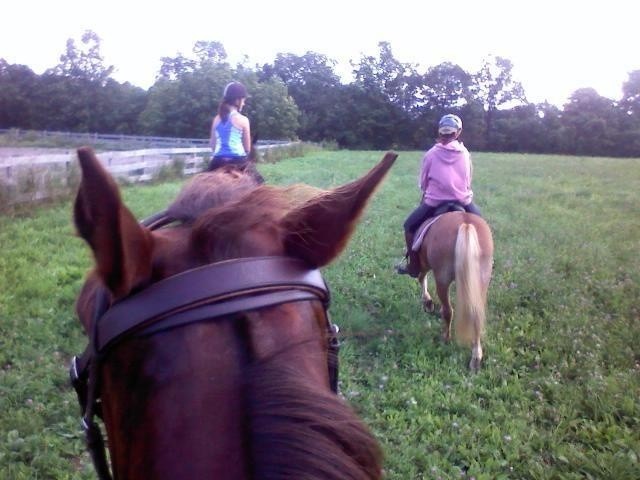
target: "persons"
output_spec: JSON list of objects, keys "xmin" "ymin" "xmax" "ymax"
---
[
  {"xmin": 208, "ymin": 81, "xmax": 265, "ymax": 185},
  {"xmin": 397, "ymin": 114, "xmax": 480, "ymax": 278}
]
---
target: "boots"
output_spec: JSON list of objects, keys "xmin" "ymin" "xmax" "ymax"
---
[{"xmin": 396, "ymin": 233, "xmax": 420, "ymax": 277}]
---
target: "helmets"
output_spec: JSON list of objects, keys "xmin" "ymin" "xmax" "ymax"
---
[
  {"xmin": 223, "ymin": 80, "xmax": 251, "ymax": 100},
  {"xmin": 438, "ymin": 115, "xmax": 463, "ymax": 138}
]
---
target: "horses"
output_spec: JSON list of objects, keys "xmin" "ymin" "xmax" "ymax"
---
[
  {"xmin": 416, "ymin": 209, "xmax": 492, "ymax": 370},
  {"xmin": 73, "ymin": 145, "xmax": 398, "ymax": 480},
  {"xmin": 211, "ymin": 132, "xmax": 260, "ymax": 178}
]
